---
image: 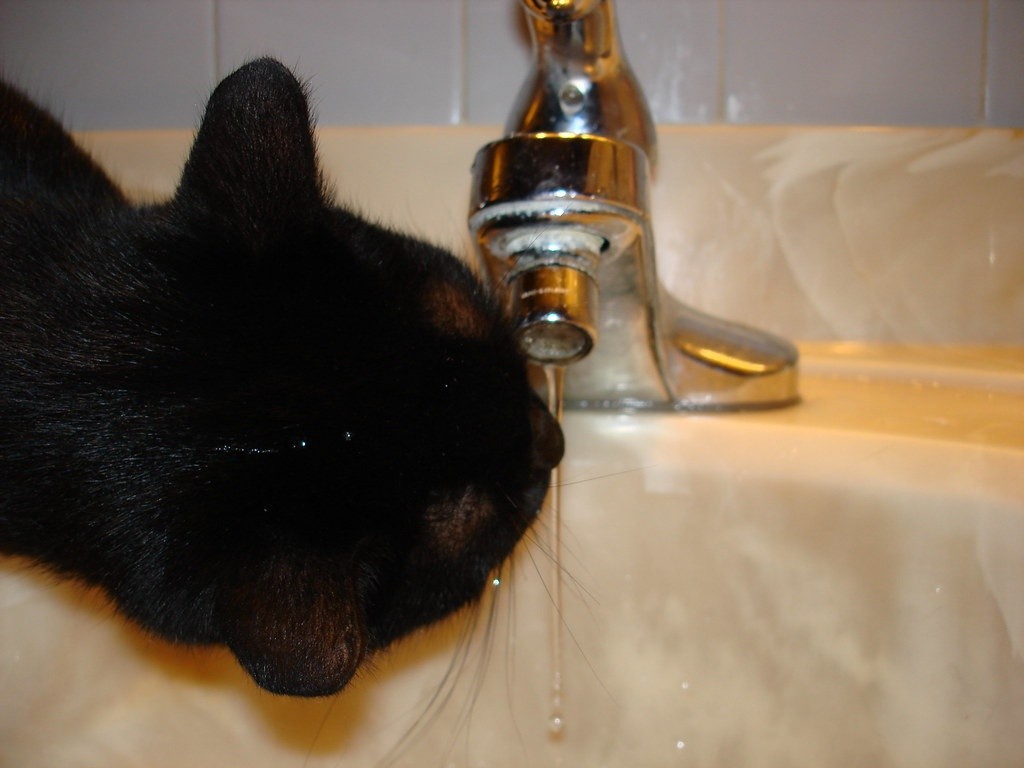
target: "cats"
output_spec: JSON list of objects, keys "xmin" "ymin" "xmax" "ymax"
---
[{"xmin": 0, "ymin": 57, "xmax": 637, "ymax": 768}]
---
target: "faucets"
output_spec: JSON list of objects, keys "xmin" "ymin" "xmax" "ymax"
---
[{"xmin": 464, "ymin": 0, "xmax": 803, "ymax": 416}]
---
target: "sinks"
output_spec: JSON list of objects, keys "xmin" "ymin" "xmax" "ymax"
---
[{"xmin": 0, "ymin": 409, "xmax": 1024, "ymax": 768}]
